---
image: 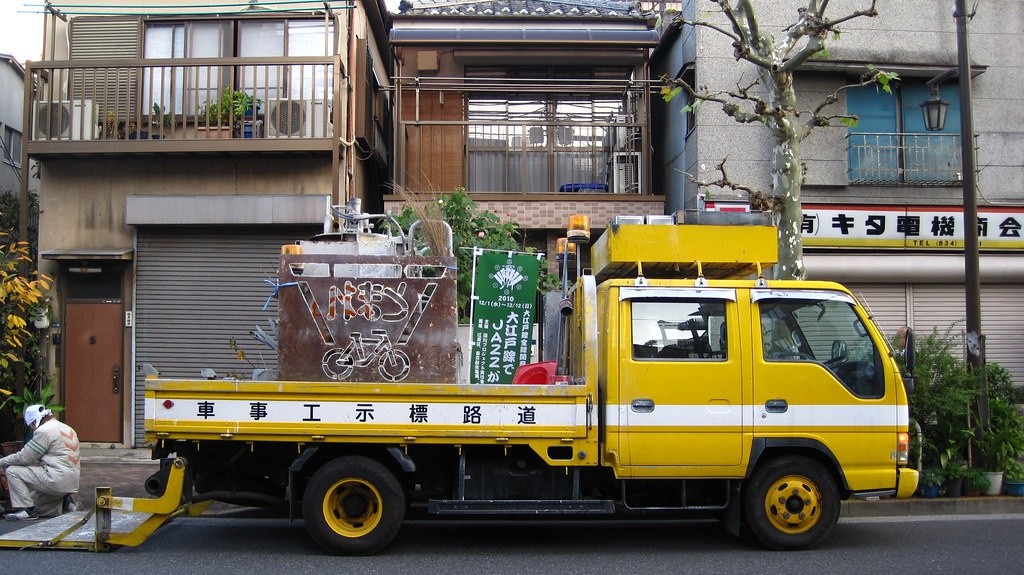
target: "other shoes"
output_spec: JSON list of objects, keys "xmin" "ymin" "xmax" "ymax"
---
[
  {"xmin": 5, "ymin": 510, "xmax": 39, "ymax": 521},
  {"xmin": 65, "ymin": 493, "xmax": 84, "ymax": 512}
]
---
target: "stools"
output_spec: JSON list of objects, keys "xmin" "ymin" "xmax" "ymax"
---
[
  {"xmin": 237, "ymin": 120, "xmax": 263, "ymax": 138},
  {"xmin": 560, "ymin": 183, "xmax": 608, "ymax": 192}
]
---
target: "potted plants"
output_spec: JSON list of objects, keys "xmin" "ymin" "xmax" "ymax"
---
[
  {"xmin": 918, "ymin": 456, "xmax": 1024, "ymax": 497},
  {"xmin": 198, "ymin": 83, "xmax": 265, "ymax": 138}
]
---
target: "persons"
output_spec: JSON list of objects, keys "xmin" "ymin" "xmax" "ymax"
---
[
  {"xmin": 0, "ymin": 405, "xmax": 80, "ymax": 521},
  {"xmin": 895, "ymin": 327, "xmax": 912, "ymax": 355}
]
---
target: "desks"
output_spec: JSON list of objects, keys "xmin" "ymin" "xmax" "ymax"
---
[{"xmin": 606, "ymin": 151, "xmax": 641, "ymax": 194}]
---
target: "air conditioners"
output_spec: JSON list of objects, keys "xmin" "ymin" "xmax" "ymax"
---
[
  {"xmin": 32, "ymin": 100, "xmax": 99, "ymax": 140},
  {"xmin": 609, "ymin": 162, "xmax": 634, "ymax": 193},
  {"xmin": 268, "ymin": 97, "xmax": 333, "ymax": 138},
  {"xmin": 612, "ymin": 114, "xmax": 635, "ymax": 152}
]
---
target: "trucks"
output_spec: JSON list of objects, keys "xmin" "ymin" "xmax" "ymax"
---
[{"xmin": 0, "ymin": 214, "xmax": 921, "ymax": 552}]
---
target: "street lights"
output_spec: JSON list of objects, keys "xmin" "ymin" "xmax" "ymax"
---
[{"xmin": 923, "ymin": 66, "xmax": 988, "ymax": 435}]
